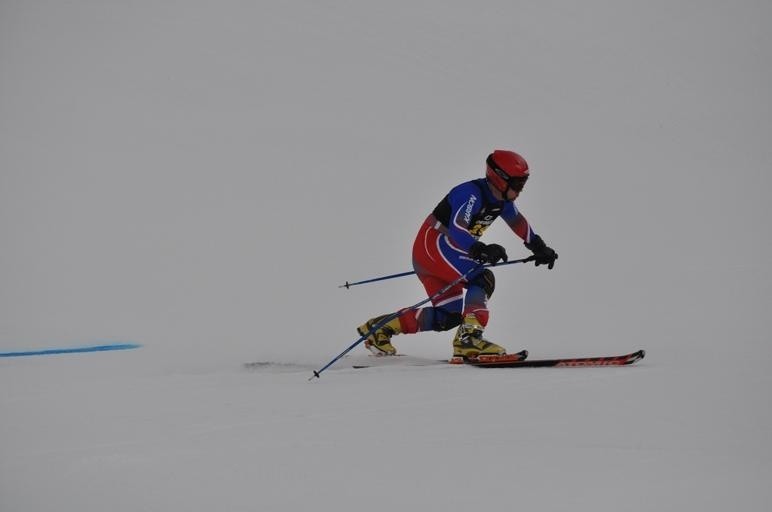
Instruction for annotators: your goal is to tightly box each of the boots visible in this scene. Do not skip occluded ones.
[453,313,505,355]
[357,312,401,354]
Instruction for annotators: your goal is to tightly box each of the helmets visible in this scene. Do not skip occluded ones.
[487,150,529,192]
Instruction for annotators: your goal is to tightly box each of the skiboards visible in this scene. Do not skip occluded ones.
[244,349,645,370]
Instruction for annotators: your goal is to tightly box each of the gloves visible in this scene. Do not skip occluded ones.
[525,234,555,270]
[470,240,507,266]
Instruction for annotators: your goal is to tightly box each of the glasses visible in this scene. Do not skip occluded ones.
[488,154,526,193]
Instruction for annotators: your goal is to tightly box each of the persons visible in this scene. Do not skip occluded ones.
[355,147,559,360]
